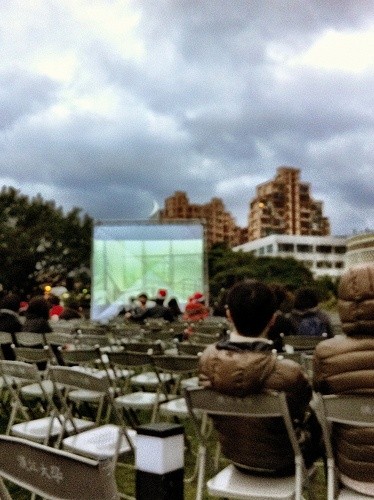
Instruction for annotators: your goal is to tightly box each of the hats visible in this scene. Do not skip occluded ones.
[195,292,205,301]
[292,289,321,315]
[159,290,167,297]
[189,297,195,303]
[20,301,28,307]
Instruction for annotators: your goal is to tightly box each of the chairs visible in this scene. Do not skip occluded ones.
[0,310,374,500]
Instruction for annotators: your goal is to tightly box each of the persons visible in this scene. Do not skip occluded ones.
[311,265,374,497]
[0,294,91,380]
[117,289,227,343]
[264,270,343,353]
[197,281,324,478]
